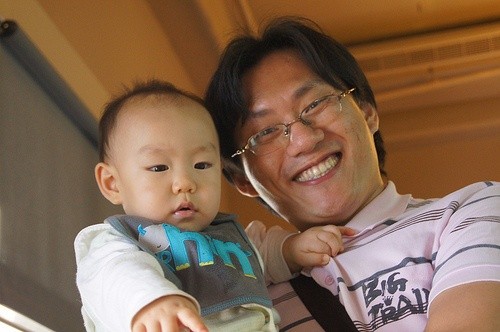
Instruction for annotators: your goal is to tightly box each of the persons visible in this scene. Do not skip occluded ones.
[202,13,500,332]
[73,78,356,332]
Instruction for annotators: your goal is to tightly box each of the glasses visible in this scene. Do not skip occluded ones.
[229,88,360,158]
